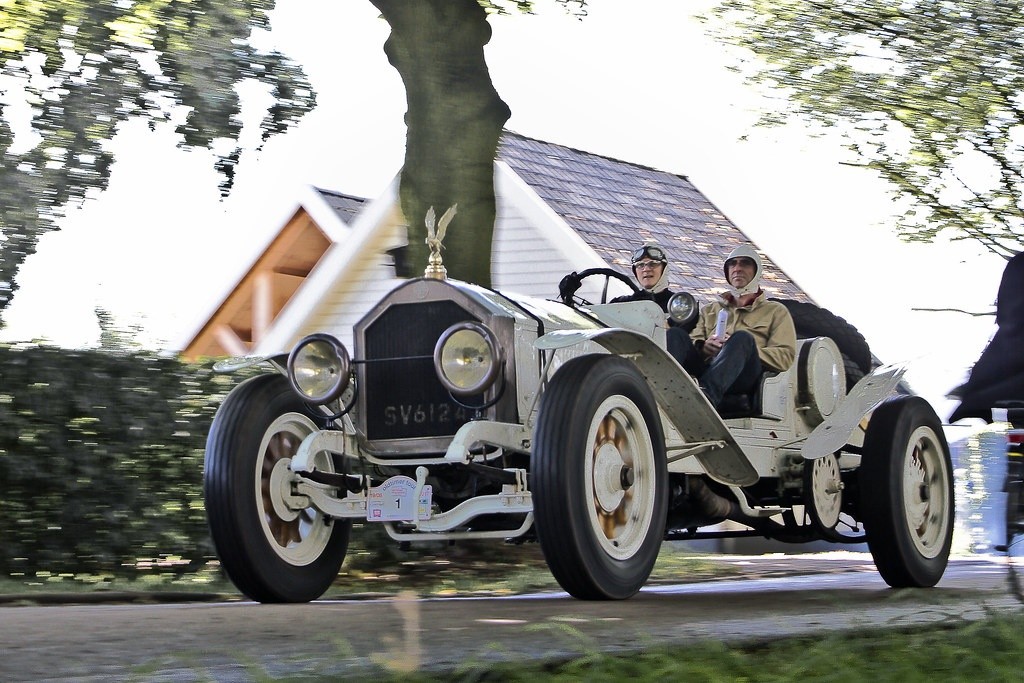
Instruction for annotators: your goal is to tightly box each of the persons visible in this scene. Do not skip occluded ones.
[996,410,1024,552]
[610,242,699,334]
[666,245,796,409]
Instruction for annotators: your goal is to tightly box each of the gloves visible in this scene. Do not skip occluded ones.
[559,272,583,298]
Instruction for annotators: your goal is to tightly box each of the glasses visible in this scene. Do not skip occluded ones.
[725,259,754,268]
[633,259,662,269]
[630,245,667,261]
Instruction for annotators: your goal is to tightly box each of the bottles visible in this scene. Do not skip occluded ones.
[716,308,728,339]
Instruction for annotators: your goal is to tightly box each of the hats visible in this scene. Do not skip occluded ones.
[631,242,671,294]
[721,245,764,296]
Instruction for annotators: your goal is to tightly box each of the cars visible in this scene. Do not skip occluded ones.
[203,265,955,601]
